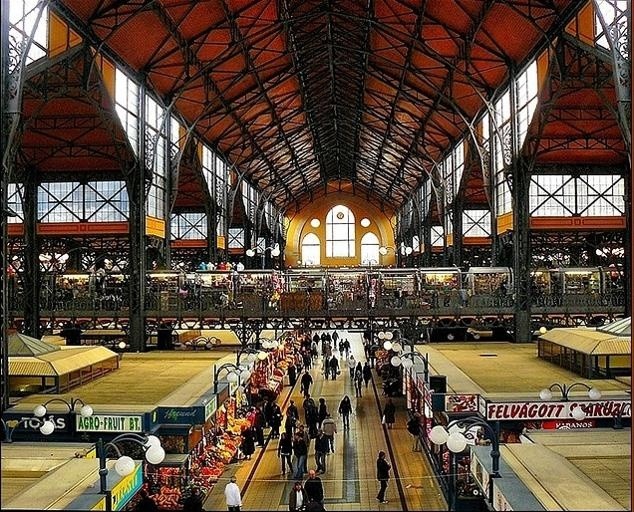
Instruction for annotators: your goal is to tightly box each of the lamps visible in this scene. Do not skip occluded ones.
[214,337,279,394]
[1,396,94,443]
[96,433,168,479]
[540,383,632,429]
[377,329,429,381]
[428,416,501,474]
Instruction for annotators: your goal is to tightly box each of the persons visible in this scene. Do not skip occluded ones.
[223,331,425,511]
[198,259,244,273]
[135,489,158,512]
[182,487,207,511]
